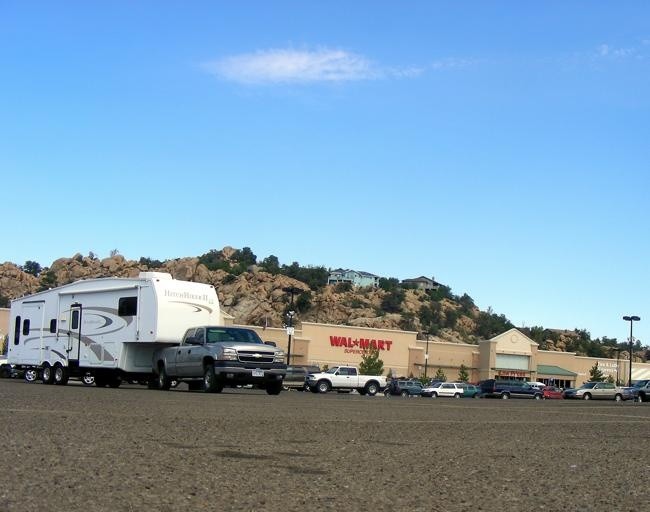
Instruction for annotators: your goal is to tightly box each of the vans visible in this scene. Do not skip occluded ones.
[283,365,319,391]
[623,380,650,402]
[463,384,481,398]
[494,380,544,400]
[421,383,464,399]
[391,380,423,397]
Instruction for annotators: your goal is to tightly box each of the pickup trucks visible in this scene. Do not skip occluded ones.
[304,366,388,395]
[152,326,287,395]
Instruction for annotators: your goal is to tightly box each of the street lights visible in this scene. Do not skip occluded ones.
[283,286,304,365]
[623,315,640,387]
[421,324,431,386]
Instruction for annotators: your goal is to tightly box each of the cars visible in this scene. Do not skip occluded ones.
[565,382,624,401]
[540,386,563,399]
